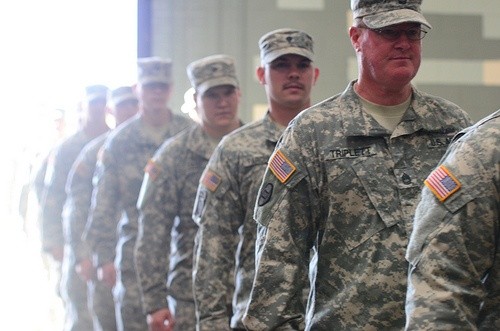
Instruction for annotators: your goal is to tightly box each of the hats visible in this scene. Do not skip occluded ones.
[351,0,432,30]
[259,29,315,63]
[186,55,240,96]
[137,57,172,86]
[112,87,139,107]
[87,85,107,103]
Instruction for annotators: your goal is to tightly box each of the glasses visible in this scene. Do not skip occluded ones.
[358,25,428,40]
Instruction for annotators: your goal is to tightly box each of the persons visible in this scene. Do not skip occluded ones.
[83,59,199,331]
[133,55,250,331]
[190,28,320,331]
[32,84,137,331]
[229,0,473,331]
[404,105,500,331]
